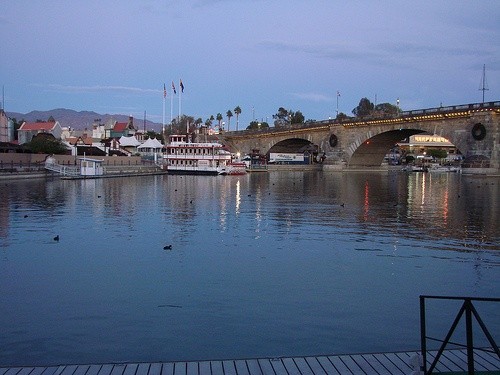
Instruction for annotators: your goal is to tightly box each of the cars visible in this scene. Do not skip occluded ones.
[0,142,129,156]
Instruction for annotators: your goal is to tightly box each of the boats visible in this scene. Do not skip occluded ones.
[162,134,248,176]
[244,148,269,172]
[384,153,463,172]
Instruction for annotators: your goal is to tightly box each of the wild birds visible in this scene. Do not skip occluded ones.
[97,195,102,198]
[53,234,60,242]
[273,184,275,185]
[292,182,296,185]
[340,202,345,207]
[189,199,193,204]
[180,176,182,178]
[163,244,173,251]
[458,194,461,198]
[247,194,251,197]
[470,182,495,188]
[23,214,29,219]
[299,179,302,182]
[268,192,271,195]
[174,189,178,191]
